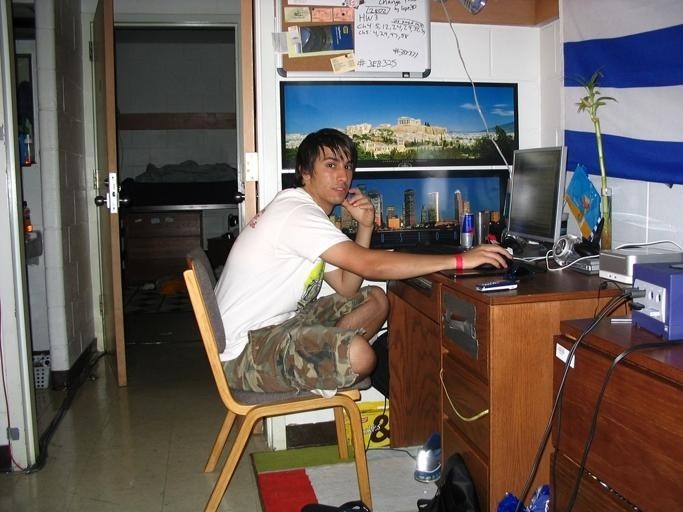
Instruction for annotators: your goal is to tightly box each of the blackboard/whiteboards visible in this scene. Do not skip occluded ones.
[272,0,431,78]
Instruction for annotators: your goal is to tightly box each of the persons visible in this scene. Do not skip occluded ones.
[206,125,514,391]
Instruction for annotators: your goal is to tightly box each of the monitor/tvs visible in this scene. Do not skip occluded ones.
[507,145,568,257]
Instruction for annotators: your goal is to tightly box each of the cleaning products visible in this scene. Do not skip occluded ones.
[18,130,25,165]
[23,200,32,229]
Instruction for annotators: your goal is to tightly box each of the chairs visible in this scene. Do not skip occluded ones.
[182,243,374,512]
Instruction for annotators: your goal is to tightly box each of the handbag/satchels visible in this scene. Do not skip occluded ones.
[301,498,374,512]
[415,452,479,512]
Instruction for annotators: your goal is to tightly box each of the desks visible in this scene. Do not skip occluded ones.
[117,203,239,288]
[385,248,633,512]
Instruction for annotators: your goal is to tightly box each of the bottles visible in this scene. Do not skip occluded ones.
[487,211,504,245]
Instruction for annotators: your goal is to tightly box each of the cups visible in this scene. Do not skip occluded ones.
[475,211,490,246]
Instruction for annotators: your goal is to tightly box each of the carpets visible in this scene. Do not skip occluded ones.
[248,434,441,511]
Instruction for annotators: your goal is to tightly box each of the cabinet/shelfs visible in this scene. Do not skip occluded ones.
[548,334,683,511]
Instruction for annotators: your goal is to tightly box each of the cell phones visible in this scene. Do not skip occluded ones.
[475,281,517,291]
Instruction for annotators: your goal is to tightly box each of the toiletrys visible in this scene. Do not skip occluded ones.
[460,214,475,252]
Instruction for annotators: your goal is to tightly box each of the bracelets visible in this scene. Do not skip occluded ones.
[455,253,464,270]
